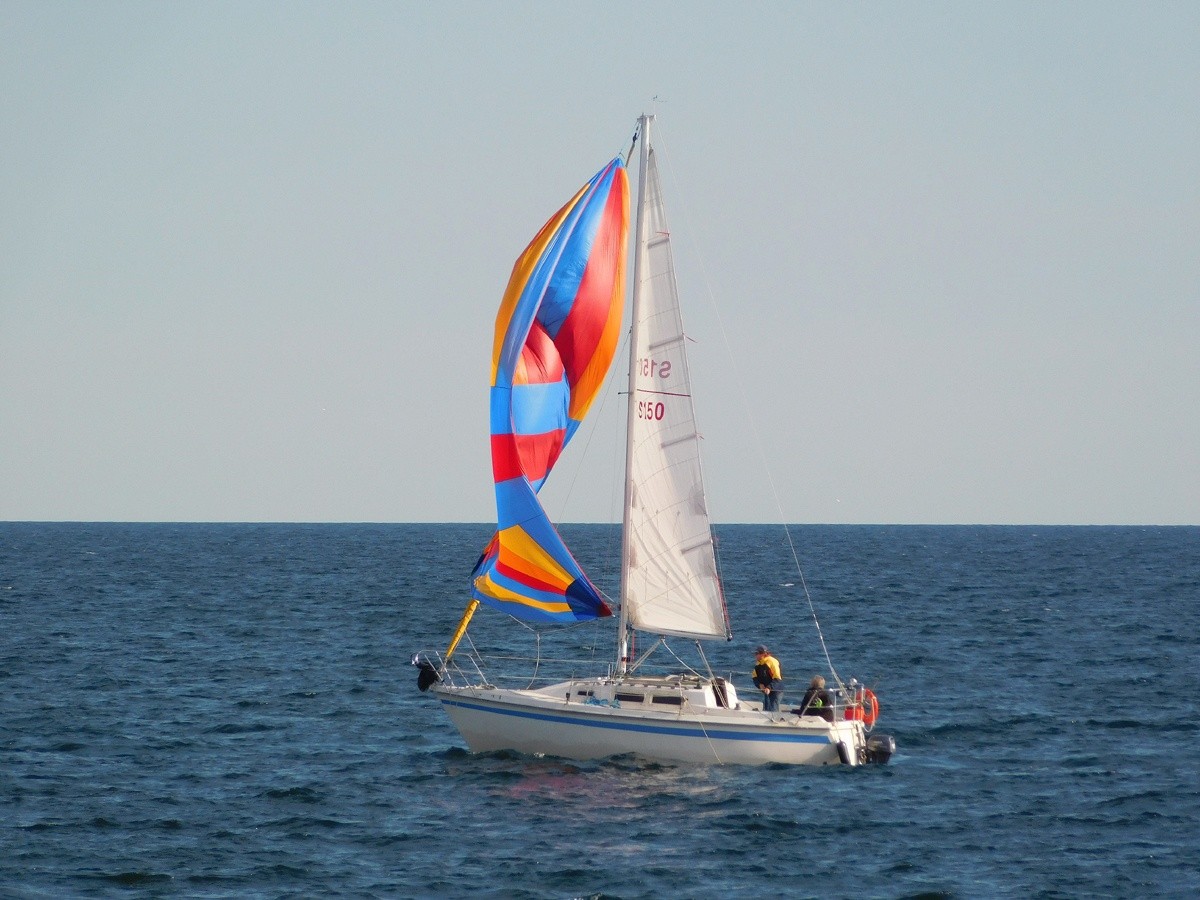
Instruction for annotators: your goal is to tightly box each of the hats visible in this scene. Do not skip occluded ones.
[751,644,767,654]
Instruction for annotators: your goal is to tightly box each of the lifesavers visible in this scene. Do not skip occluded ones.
[856,689,878,724]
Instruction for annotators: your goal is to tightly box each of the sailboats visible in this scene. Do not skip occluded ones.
[411,111,897,769]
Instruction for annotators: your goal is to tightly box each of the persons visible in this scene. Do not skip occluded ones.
[801,676,833,722]
[752,645,784,712]
